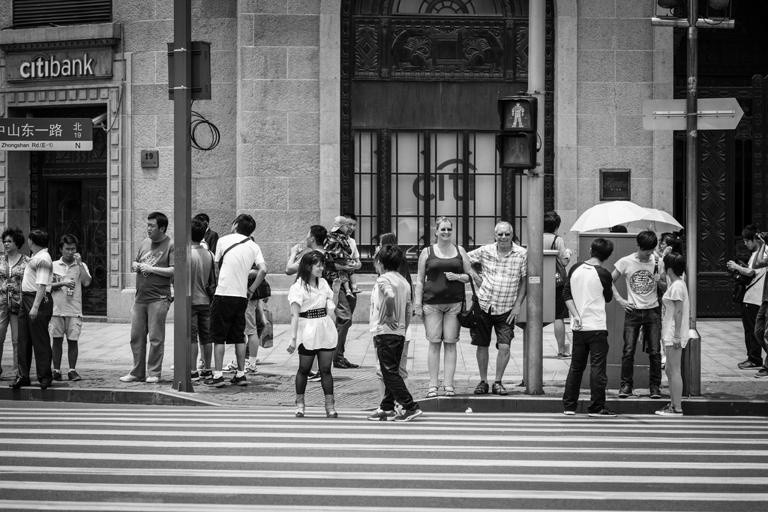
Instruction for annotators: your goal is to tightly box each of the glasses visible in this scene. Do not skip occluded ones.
[497,233,511,236]
[439,228,452,231]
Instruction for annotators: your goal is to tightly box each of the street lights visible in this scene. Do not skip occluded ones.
[657,0,729,399]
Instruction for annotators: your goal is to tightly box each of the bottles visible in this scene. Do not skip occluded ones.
[67,278,75,297]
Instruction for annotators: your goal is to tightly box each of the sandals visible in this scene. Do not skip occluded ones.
[426,386,440,398]
[443,385,457,396]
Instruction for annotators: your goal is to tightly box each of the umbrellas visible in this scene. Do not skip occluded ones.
[625,199,685,236]
[567,195,646,235]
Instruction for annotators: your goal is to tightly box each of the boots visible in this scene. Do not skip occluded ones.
[324,394,339,418]
[293,392,305,417]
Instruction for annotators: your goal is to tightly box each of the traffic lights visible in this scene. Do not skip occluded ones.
[496,96,537,169]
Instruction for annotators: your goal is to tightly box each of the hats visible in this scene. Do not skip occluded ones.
[331,215,347,233]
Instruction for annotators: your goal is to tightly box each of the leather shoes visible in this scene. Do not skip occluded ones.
[40,379,52,390]
[8,378,31,388]
[333,358,359,368]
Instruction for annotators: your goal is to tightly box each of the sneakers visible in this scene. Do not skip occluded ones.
[473,381,490,394]
[754,366,768,378]
[306,370,322,382]
[491,383,508,395]
[395,402,423,422]
[367,408,399,421]
[198,368,213,380]
[50,370,62,381]
[618,383,633,397]
[737,360,764,369]
[221,358,257,373]
[67,370,81,382]
[203,376,225,387]
[146,376,162,382]
[588,407,617,417]
[563,408,576,415]
[119,374,145,383]
[189,371,200,381]
[653,403,684,416]
[229,373,247,386]
[649,385,662,398]
[346,289,356,298]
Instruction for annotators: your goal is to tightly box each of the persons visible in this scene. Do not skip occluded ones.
[8,229,55,391]
[562,237,619,417]
[541,209,573,361]
[48,232,92,384]
[412,212,473,398]
[608,229,662,397]
[651,251,692,418]
[0,228,30,383]
[657,236,687,296]
[466,219,528,396]
[750,232,768,378]
[723,224,765,370]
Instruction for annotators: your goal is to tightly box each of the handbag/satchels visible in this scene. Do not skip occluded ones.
[256,302,275,348]
[655,274,670,315]
[731,268,752,285]
[550,235,567,288]
[247,277,272,301]
[456,272,483,329]
[206,250,218,300]
[732,285,745,303]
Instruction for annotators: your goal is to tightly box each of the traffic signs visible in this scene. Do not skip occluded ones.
[0,117,95,152]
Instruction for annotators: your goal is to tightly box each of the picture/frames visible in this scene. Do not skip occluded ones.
[599,167,633,202]
